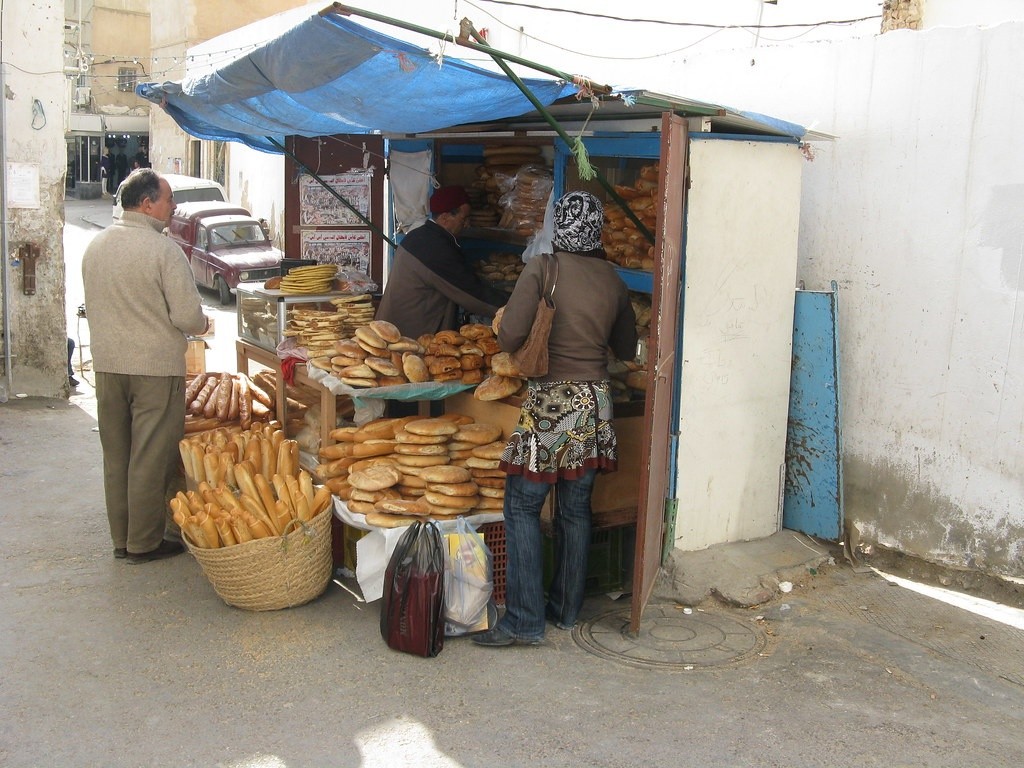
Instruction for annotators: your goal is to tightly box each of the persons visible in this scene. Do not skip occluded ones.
[130,161,140,173]
[91,140,100,182]
[102,147,150,196]
[471,186,637,648]
[80,168,210,564]
[372,186,509,416]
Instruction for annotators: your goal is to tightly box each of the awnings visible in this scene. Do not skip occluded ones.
[136,2,656,251]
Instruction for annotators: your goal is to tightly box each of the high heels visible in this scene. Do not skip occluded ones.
[544,606,564,630]
[471,626,540,646]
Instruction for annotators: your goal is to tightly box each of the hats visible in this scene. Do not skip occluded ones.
[430,185,469,212]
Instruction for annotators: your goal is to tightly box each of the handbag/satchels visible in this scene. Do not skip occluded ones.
[508,254,561,377]
[380,519,444,658]
[433,515,499,636]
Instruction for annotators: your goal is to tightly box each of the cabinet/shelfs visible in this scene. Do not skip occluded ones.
[427,137,667,396]
[235,339,430,494]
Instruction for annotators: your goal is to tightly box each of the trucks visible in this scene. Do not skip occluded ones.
[166,200,286,306]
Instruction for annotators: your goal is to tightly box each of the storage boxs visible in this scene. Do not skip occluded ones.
[237,282,360,354]
[444,390,645,606]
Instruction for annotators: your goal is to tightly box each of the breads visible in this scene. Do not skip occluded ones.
[457,146,552,235]
[169,304,542,550]
[601,293,652,402]
[479,249,528,294]
[597,160,660,272]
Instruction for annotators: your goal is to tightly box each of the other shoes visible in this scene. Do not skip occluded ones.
[112,538,183,564]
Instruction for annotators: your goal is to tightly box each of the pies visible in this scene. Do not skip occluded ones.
[240,265,376,360]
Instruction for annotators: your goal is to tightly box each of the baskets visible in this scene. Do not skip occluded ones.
[180,482,334,612]
